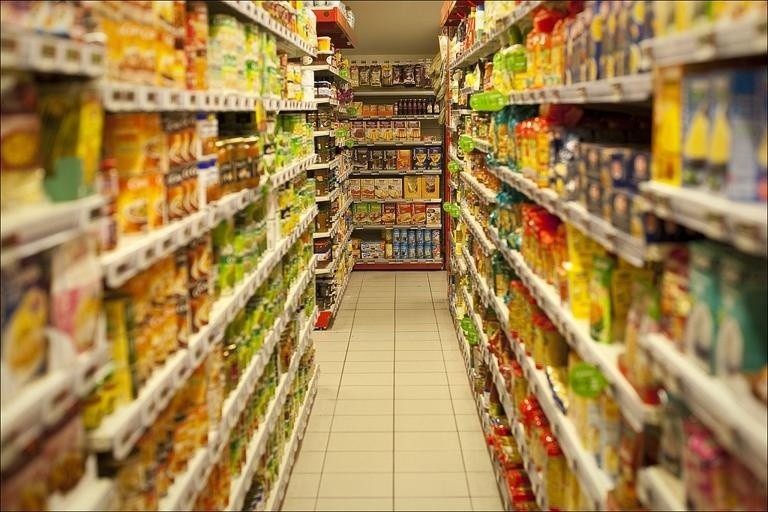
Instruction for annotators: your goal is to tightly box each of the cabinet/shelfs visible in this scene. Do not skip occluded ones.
[440,0,768,512]
[313,0,356,331]
[0,0,321,511]
[346,63,446,271]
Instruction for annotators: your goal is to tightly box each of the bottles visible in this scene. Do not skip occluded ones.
[314,80,337,309]
[394,96,440,115]
[198,115,260,208]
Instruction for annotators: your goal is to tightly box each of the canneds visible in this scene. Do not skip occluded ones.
[215,136,261,196]
